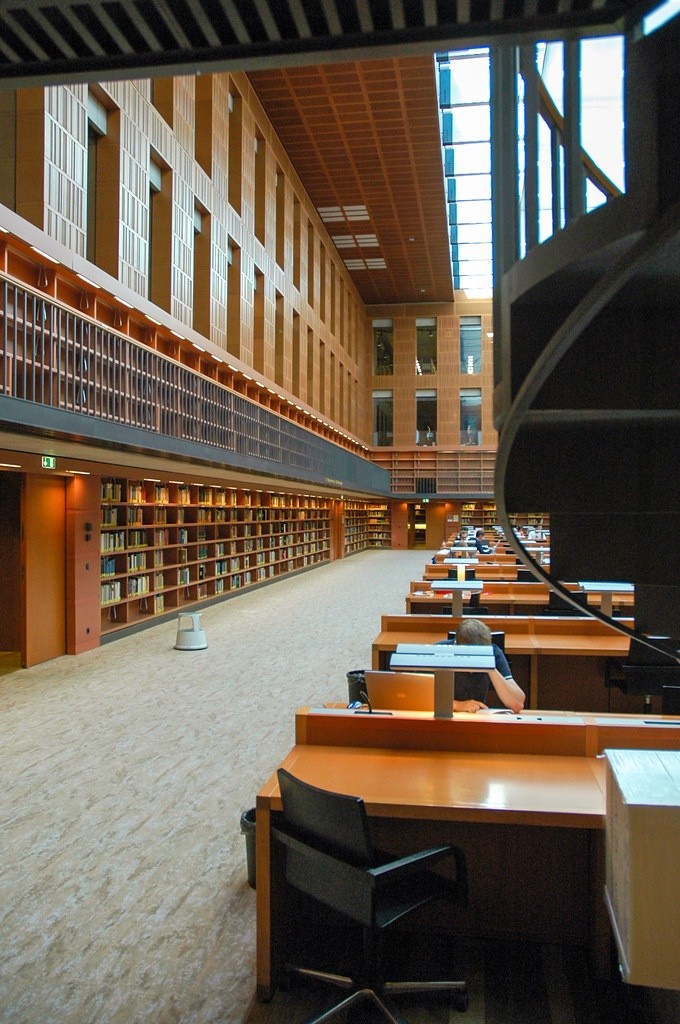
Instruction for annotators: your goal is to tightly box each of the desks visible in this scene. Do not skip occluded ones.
[442,531,550,547]
[405,547,636,615]
[253,614,680,1024]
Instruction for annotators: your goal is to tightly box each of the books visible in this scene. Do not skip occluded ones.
[100,483,389,607]
[460,504,543,524]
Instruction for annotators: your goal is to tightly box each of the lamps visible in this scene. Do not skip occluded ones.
[578,578,637,619]
[430,580,485,618]
[387,639,499,718]
[441,558,481,581]
[449,545,478,558]
[535,528,550,539]
[525,546,551,565]
[454,526,535,543]
[519,540,537,547]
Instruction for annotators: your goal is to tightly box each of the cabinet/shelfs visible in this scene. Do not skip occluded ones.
[93,475,253,639]
[368,446,499,498]
[413,505,427,542]
[603,747,680,996]
[253,483,335,584]
[340,498,392,557]
[459,502,549,531]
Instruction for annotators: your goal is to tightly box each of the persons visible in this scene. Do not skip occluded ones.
[527,524,547,540]
[454,527,493,554]
[514,525,523,536]
[434,619,527,713]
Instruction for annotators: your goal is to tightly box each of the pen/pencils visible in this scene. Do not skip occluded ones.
[517,717,541,720]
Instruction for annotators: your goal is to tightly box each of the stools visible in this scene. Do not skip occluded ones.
[170,609,210,651]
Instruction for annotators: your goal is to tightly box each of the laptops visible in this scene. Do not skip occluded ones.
[365,669,434,711]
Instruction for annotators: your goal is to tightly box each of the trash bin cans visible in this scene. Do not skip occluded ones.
[346,670,372,704]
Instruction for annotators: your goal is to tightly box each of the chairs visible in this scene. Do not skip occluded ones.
[266,766,472,1024]
[598,624,680,723]
